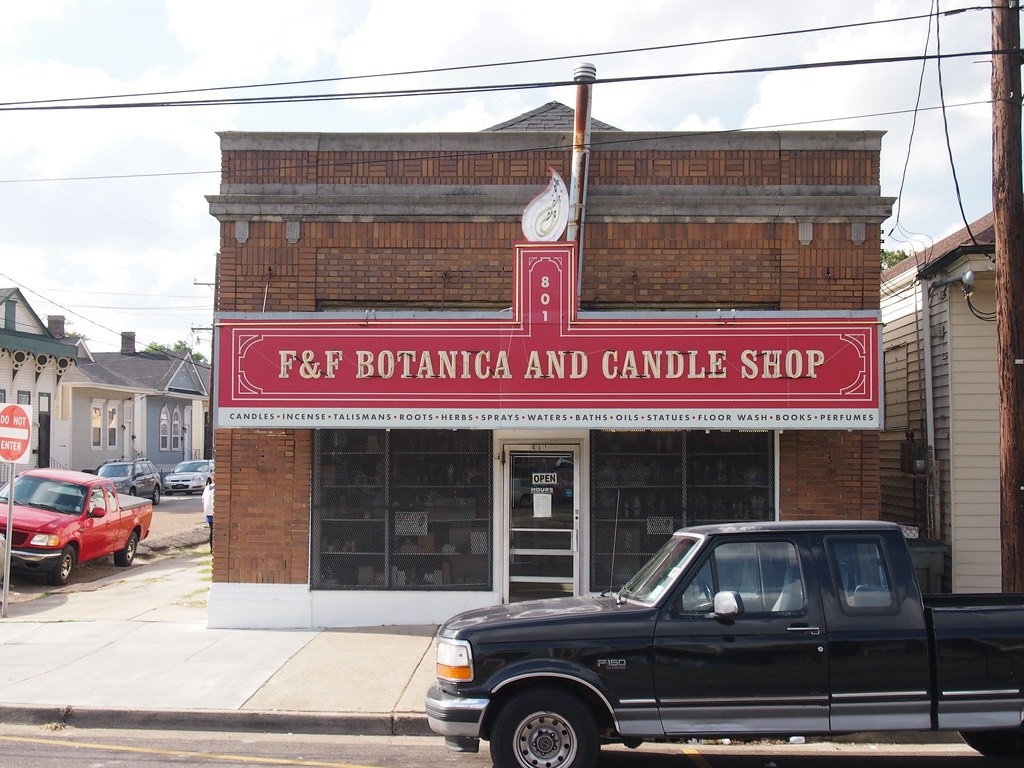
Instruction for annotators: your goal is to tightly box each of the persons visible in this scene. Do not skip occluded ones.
[201,471,215,553]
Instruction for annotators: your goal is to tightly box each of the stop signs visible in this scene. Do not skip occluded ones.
[0,404,31,461]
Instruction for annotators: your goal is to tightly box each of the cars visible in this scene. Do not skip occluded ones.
[163,460,214,496]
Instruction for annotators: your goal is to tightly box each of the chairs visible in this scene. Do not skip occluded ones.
[769,566,804,611]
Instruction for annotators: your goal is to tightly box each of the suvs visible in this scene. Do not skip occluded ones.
[98,458,162,506]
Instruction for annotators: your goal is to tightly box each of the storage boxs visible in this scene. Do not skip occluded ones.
[449,528,487,552]
[358,567,375,585]
[425,498,475,520]
[419,532,435,553]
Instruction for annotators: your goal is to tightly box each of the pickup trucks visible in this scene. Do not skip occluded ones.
[0,468,153,586]
[423,520,1024,768]
